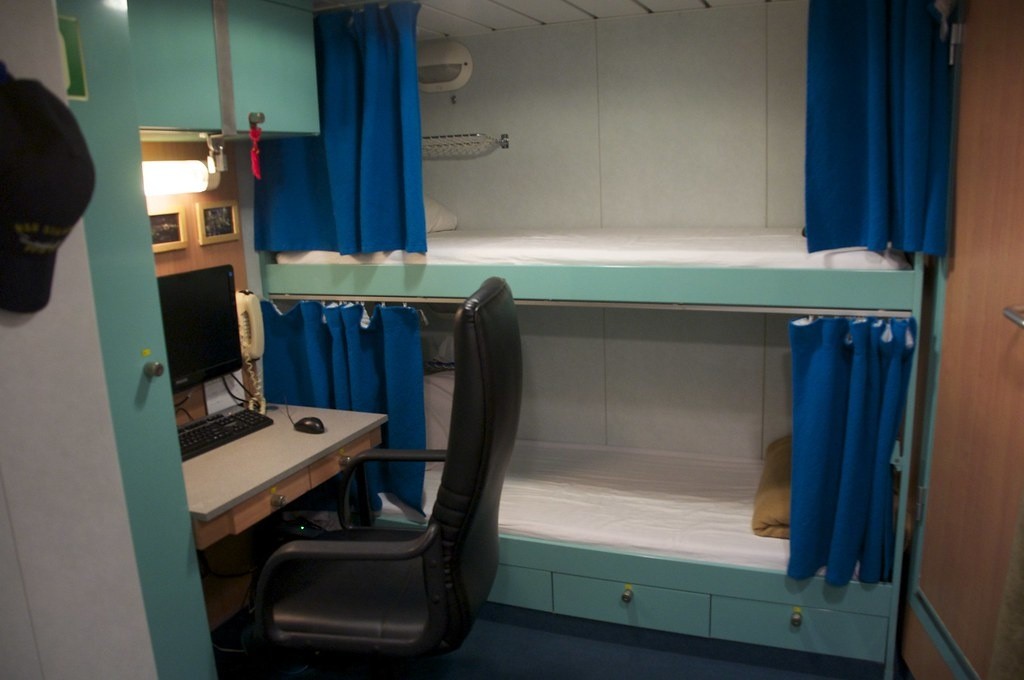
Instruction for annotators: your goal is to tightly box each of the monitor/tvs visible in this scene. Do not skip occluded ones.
[159,264,243,395]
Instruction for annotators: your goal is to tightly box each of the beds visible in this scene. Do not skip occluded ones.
[257,234,929,679]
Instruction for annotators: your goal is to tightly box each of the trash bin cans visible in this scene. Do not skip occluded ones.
[211,604,271,680]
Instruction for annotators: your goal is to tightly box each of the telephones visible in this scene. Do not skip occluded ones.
[235,290,265,362]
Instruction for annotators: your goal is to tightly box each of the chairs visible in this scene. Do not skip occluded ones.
[252,275,525,680]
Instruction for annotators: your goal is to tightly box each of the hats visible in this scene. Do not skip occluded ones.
[0,63,96,313]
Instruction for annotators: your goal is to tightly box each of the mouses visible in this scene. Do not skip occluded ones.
[293,417,324,434]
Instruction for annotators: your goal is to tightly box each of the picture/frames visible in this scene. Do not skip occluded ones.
[147,205,188,255]
[196,198,241,246]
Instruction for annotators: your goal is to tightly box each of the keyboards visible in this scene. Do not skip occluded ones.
[178,405,273,461]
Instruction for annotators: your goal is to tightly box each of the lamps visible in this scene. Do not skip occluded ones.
[141,130,230,197]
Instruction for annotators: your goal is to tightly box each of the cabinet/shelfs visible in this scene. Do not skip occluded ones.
[127,0,320,143]
[891,0,1024,679]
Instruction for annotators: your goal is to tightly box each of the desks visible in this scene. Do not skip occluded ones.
[178,398,391,636]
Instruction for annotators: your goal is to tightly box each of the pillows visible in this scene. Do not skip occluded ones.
[423,197,458,231]
[424,370,455,470]
